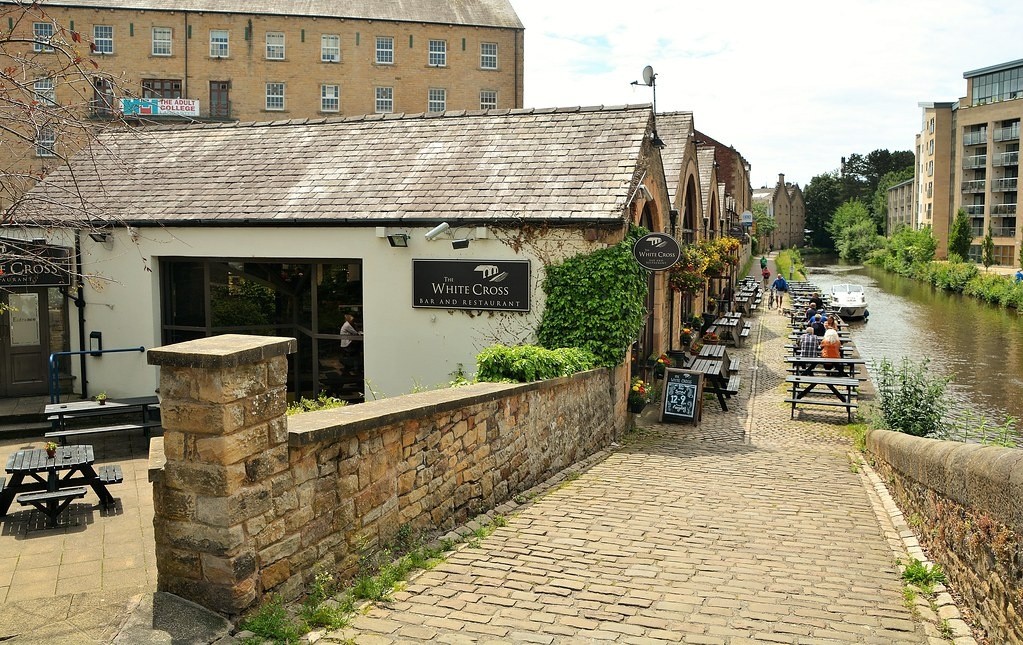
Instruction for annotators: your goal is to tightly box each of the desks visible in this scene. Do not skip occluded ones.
[0,445,114,527]
[736,292,753,297]
[790,303,832,307]
[690,359,723,376]
[44,396,162,446]
[742,286,756,292]
[785,375,860,387]
[724,312,742,318]
[792,298,832,303]
[788,322,849,327]
[787,315,839,320]
[790,308,841,314]
[792,328,850,334]
[713,317,738,327]
[786,356,865,364]
[787,334,852,342]
[784,344,853,352]
[733,297,748,302]
[699,345,726,358]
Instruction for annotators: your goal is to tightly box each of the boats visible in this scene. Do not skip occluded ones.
[829,284,868,317]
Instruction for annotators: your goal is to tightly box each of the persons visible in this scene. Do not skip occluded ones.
[805,292,839,336]
[820,328,844,377]
[760,256,771,292]
[770,273,789,309]
[339,314,363,357]
[798,327,820,376]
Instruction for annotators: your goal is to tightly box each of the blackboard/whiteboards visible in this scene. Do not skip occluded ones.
[659,367,703,423]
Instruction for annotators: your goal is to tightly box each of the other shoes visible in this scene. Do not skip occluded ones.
[765,288,768,291]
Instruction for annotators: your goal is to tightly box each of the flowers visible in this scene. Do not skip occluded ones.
[96,393,107,400]
[692,313,704,324]
[669,234,740,307]
[629,379,654,403]
[656,353,672,372]
[43,441,57,450]
[682,328,694,340]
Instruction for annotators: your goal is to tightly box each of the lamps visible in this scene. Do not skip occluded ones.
[88,232,111,242]
[425,221,450,241]
[649,130,666,149]
[452,238,469,249]
[713,160,720,167]
[691,139,707,146]
[387,232,410,247]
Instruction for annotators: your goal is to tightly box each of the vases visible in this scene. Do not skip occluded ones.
[694,327,700,331]
[654,373,665,379]
[47,450,55,457]
[710,307,715,311]
[682,340,690,346]
[630,402,645,414]
[99,400,106,405]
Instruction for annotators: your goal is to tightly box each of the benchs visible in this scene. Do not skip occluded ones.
[684,276,763,395]
[784,279,867,408]
[47,407,157,421]
[17,487,87,505]
[45,421,161,441]
[99,465,123,485]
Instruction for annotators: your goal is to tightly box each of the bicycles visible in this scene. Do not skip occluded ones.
[768,289,777,310]
